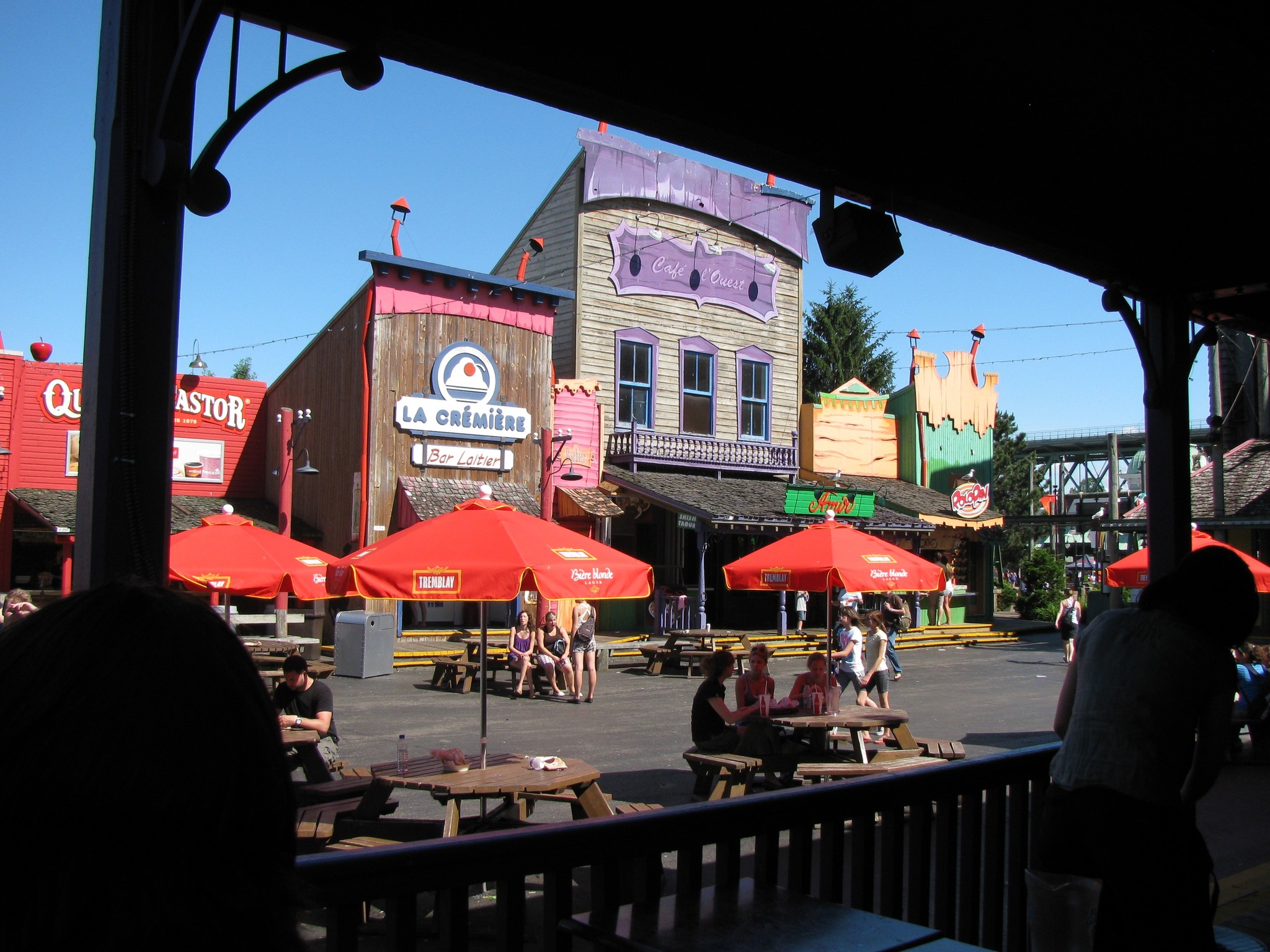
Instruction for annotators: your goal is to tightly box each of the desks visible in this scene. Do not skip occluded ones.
[281,723,336,782]
[240,638,302,692]
[334,753,616,838]
[444,636,568,691]
[642,628,772,679]
[570,876,942,952]
[898,936,998,952]
[690,703,927,803]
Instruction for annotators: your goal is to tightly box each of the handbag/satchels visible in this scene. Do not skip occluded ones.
[1010,574,1016,581]
[1059,599,1076,630]
[553,638,566,657]
[1004,572,1008,579]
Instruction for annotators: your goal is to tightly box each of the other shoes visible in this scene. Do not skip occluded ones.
[894,673,903,680]
[568,698,581,704]
[1062,655,1069,663]
[874,726,884,736]
[553,691,565,696]
[795,630,807,635]
[515,689,523,696]
[569,692,583,697]
[529,694,536,699]
[584,698,592,703]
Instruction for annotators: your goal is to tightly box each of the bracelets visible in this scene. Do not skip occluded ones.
[752,703,760,712]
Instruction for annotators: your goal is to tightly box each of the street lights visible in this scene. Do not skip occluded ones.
[528,426,584,629]
[275,407,320,638]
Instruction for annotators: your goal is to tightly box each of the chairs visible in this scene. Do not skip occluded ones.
[647,599,689,633]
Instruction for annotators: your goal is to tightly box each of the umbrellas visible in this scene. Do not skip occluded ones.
[324,485,654,769]
[166,503,357,635]
[1102,521,1270,594]
[723,509,946,714]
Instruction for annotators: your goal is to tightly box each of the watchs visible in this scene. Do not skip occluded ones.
[295,716,302,726]
[890,608,894,613]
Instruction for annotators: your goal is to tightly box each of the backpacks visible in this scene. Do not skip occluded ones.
[891,594,912,632]
[575,605,595,644]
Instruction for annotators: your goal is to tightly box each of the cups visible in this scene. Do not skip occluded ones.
[173,447,180,474]
[824,685,841,715]
[707,623,711,632]
[812,692,823,715]
[184,461,204,478]
[199,454,221,479]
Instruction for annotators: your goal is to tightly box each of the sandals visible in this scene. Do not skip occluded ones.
[864,738,873,742]
[874,740,883,744]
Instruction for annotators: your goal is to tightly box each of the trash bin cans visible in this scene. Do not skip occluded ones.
[264,595,326,660]
[333,609,395,679]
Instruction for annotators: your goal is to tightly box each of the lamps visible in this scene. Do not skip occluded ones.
[801,480,825,500]
[834,483,858,505]
[864,485,888,508]
[695,228,723,256]
[754,244,777,276]
[188,338,208,375]
[636,212,664,243]
[811,188,905,280]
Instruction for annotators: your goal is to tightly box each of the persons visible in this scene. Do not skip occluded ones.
[408,600,427,630]
[688,588,910,791]
[0,587,41,626]
[993,565,1270,952]
[270,655,340,783]
[0,578,302,952]
[508,609,536,699]
[568,600,597,704]
[536,611,583,697]
[928,552,953,626]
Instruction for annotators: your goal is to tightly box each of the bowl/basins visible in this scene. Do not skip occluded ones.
[442,761,470,772]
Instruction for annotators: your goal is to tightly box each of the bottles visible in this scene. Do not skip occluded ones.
[528,756,544,771]
[759,694,771,717]
[803,682,812,708]
[397,734,409,771]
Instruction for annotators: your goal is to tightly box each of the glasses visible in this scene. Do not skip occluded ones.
[838,613,848,618]
[751,646,765,653]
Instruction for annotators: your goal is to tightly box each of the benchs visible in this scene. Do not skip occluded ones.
[251,654,338,697]
[682,728,966,832]
[292,755,665,924]
[429,652,576,695]
[638,641,778,680]
